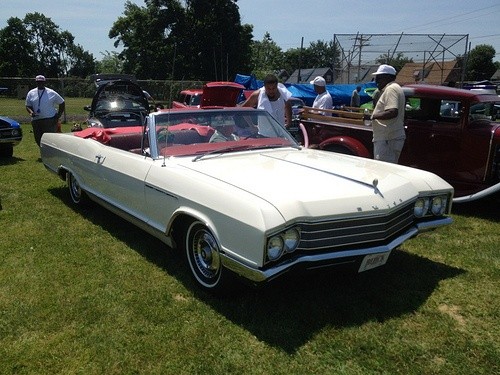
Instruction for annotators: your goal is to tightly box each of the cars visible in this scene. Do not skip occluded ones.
[83,79,158,129]
[171,81,259,141]
[455,80,500,109]
[0,114,23,159]
[284,83,500,211]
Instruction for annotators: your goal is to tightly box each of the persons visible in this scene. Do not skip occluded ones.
[238,74,292,139]
[141,87,155,105]
[447,80,456,88]
[326,72,331,82]
[24,75,65,160]
[370,64,406,164]
[309,76,333,122]
[372,87,382,107]
[350,86,361,118]
[209,115,240,145]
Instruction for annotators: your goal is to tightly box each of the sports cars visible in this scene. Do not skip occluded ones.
[40,105,455,296]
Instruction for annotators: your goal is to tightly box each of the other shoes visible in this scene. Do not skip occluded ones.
[38,157,42,161]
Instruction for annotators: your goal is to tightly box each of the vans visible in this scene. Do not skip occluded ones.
[90,73,136,86]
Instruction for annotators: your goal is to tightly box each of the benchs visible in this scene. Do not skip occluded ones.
[105,129,207,151]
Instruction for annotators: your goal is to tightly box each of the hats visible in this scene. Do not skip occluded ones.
[309,76,326,86]
[35,75,46,81]
[371,64,397,76]
